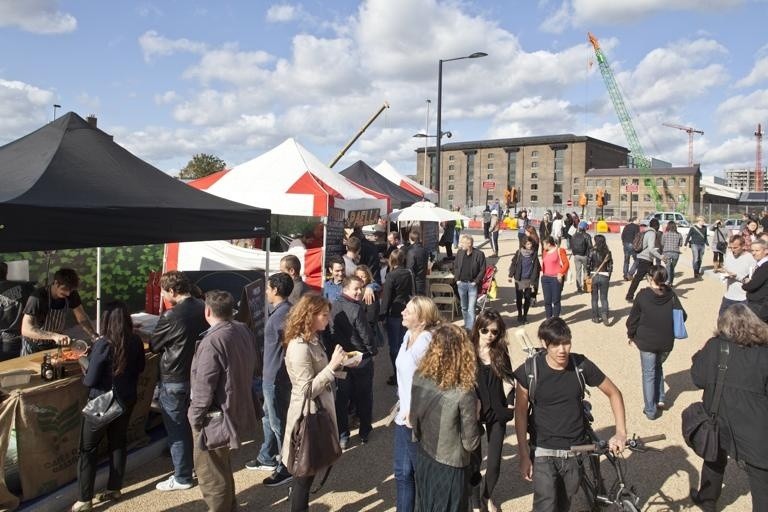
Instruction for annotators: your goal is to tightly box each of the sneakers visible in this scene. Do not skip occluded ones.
[601,313,609,326]
[263,462,293,487]
[156,475,193,490]
[245,459,278,472]
[340,436,351,450]
[359,438,369,445]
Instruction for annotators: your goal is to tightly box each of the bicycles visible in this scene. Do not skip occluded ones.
[570,427,666,512]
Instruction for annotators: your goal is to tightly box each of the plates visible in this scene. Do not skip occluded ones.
[339,350,364,365]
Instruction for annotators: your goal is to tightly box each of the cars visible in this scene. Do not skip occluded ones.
[724,219,745,230]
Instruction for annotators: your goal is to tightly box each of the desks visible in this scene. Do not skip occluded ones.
[423,271,457,283]
[1,341,177,507]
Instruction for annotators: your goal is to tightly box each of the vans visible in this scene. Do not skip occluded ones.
[640,212,690,227]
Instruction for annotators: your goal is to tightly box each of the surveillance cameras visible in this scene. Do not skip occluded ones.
[448,133,452,138]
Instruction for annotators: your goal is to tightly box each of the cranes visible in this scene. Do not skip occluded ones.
[661,121,704,168]
[754,123,764,191]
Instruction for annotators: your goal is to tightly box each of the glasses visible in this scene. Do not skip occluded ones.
[646,273,652,279]
[478,327,498,335]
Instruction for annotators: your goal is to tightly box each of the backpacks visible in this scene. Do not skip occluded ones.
[632,229,657,254]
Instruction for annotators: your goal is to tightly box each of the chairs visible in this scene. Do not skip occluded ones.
[430,283,462,323]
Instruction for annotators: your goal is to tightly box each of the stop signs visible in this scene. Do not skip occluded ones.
[567,200,572,207]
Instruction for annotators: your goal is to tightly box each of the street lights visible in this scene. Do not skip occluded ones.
[412,50,489,211]
[53,103,61,121]
[421,97,432,185]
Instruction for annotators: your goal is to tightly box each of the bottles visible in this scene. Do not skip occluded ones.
[40,352,62,380]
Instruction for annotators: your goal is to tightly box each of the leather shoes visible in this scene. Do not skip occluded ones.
[95,489,123,502]
[65,500,94,512]
[689,488,714,512]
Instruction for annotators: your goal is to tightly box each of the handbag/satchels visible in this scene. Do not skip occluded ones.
[672,308,688,338]
[583,278,593,293]
[286,382,343,478]
[82,386,125,434]
[681,401,720,463]
[716,242,726,253]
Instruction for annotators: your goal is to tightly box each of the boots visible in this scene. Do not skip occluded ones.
[713,260,718,273]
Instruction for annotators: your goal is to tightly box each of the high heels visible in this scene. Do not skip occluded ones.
[481,494,498,512]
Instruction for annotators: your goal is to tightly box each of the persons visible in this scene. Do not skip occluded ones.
[245,271,295,488]
[67,303,147,512]
[19,266,102,355]
[146,268,207,493]
[182,285,259,512]
[0,260,36,362]
[279,206,767,512]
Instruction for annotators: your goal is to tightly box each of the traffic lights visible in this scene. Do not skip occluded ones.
[507,191,510,203]
[513,189,517,202]
[600,191,605,206]
